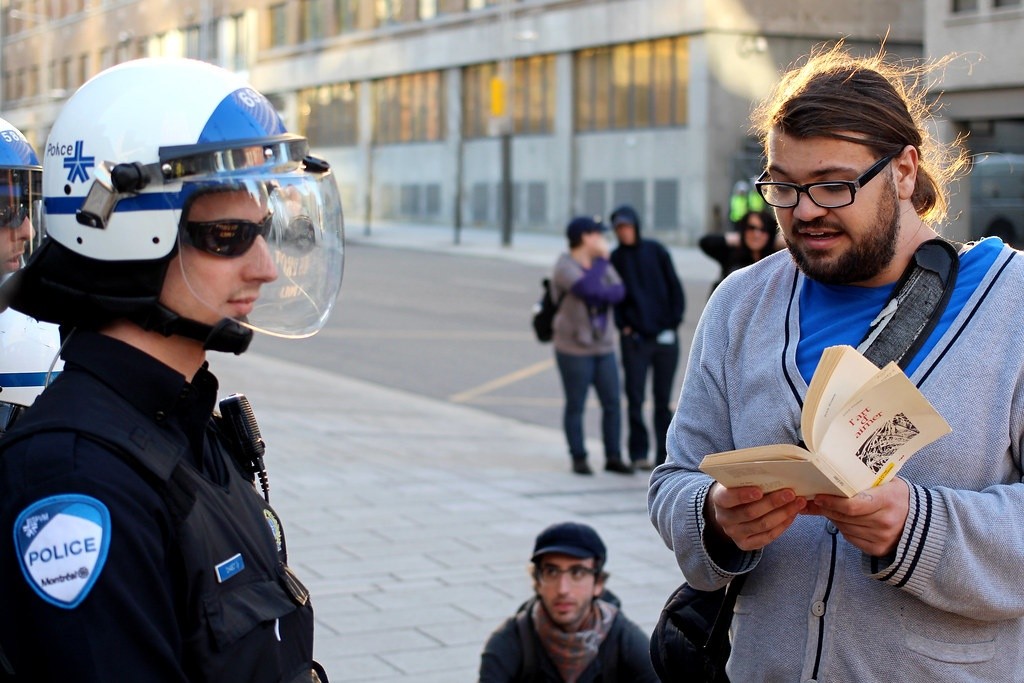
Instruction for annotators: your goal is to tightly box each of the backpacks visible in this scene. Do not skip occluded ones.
[532,279,565,343]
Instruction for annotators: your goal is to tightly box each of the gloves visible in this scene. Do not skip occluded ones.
[657,329,676,344]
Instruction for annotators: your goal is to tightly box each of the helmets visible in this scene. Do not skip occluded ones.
[42,59,331,262]
[0,117,43,200]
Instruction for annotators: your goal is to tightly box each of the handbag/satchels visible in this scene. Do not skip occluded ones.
[650,581,731,683]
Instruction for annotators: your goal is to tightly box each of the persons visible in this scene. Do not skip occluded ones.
[479,522,660,683]
[609,207,685,472]
[730,175,765,223]
[647,56,1024,683]
[0,118,43,287]
[551,217,635,476]
[0,56,347,683]
[699,212,788,280]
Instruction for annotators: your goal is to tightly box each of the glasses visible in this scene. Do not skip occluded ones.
[2,203,27,230]
[748,225,768,233]
[755,154,891,208]
[179,217,316,259]
[539,564,596,579]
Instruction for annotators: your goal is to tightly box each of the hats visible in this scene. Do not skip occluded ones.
[736,180,749,191]
[613,212,635,228]
[567,217,608,239]
[532,522,607,567]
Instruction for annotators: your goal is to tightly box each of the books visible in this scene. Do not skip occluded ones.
[699,345,954,500]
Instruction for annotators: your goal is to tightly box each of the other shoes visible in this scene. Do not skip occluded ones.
[605,460,634,475]
[630,447,650,461]
[574,460,593,477]
